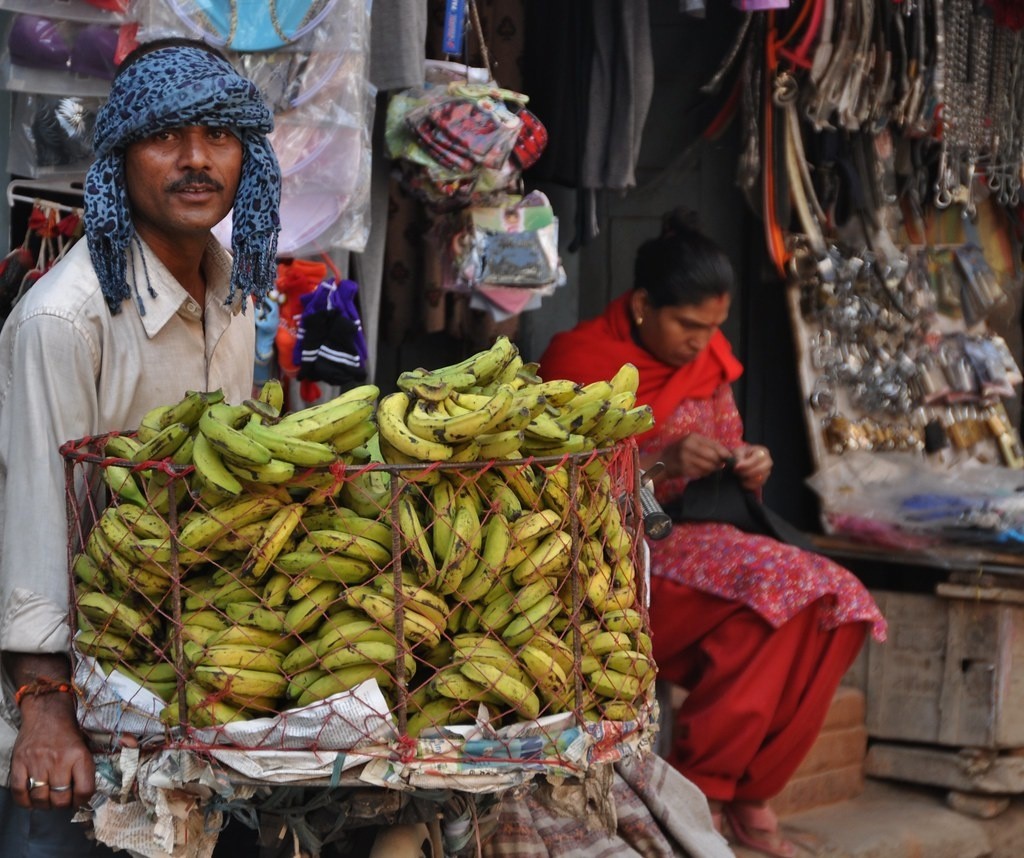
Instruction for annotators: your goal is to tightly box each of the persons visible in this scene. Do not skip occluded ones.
[0,38,283,858]
[540,224,888,858]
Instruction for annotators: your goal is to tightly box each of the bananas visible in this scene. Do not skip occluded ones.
[73,333,659,743]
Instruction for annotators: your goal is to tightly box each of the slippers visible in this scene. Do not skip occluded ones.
[713,813,722,835]
[724,799,797,858]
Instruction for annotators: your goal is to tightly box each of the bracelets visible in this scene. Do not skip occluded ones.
[15,669,73,707]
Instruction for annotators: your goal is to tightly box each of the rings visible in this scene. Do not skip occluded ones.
[28,777,47,791]
[50,784,71,792]
[758,450,765,458]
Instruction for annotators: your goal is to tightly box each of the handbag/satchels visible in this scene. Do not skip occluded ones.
[253,259,367,384]
